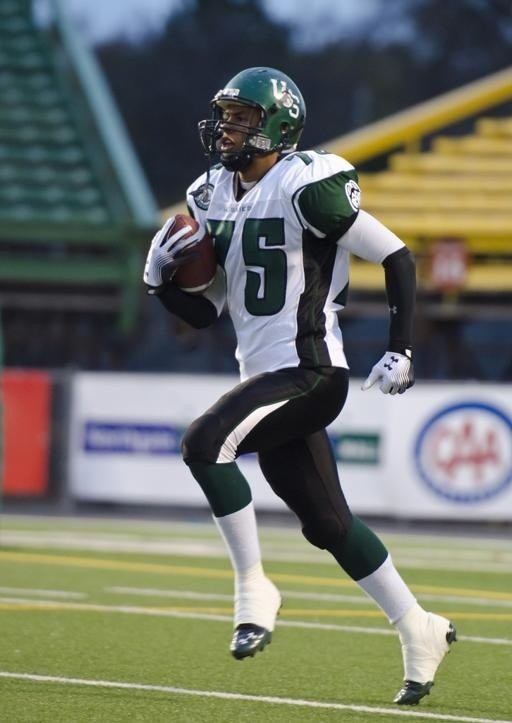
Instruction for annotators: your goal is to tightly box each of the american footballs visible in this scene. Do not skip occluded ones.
[164,213,217,296]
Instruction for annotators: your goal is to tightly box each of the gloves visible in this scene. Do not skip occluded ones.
[360,349,414,395]
[142,217,201,296]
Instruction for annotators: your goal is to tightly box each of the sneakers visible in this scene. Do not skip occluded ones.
[393,617,456,705]
[229,583,283,661]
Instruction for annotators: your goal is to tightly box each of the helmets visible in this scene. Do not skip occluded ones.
[197,67,308,172]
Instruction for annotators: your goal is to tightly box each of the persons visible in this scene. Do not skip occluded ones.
[140,65,460,706]
